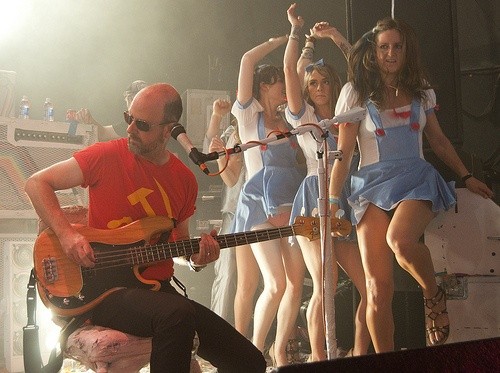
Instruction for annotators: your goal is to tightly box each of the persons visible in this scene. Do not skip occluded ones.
[23,82,268,373]
[66,79,150,139]
[205,83,247,330]
[230,26,317,369]
[282,0,371,364]
[327,17,494,357]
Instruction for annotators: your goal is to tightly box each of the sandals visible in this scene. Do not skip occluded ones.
[424,286,450,347]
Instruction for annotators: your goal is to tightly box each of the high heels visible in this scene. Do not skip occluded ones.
[269,343,290,368]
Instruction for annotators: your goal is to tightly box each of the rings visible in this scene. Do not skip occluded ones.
[206,252,211,255]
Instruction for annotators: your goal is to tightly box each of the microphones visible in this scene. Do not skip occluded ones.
[169,123,210,175]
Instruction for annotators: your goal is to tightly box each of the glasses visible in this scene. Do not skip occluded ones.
[123,111,176,131]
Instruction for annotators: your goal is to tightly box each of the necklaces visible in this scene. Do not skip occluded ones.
[373,75,401,97]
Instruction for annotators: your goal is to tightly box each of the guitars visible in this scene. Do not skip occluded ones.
[33,209,351,317]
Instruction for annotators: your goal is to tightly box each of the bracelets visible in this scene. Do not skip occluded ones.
[306,36,317,48]
[327,197,343,206]
[300,47,314,61]
[461,172,475,184]
[288,35,300,42]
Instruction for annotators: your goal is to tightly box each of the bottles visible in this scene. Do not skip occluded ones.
[20,96,30,120]
[43,97,54,122]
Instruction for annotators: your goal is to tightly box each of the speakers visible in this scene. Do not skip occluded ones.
[270,336,500,373]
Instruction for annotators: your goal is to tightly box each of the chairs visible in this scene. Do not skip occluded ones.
[38,205,203,373]
